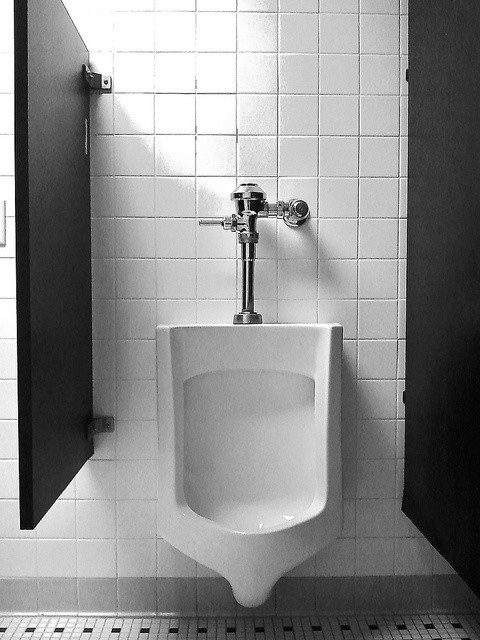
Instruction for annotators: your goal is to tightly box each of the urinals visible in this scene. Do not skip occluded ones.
[156,323,342,610]
